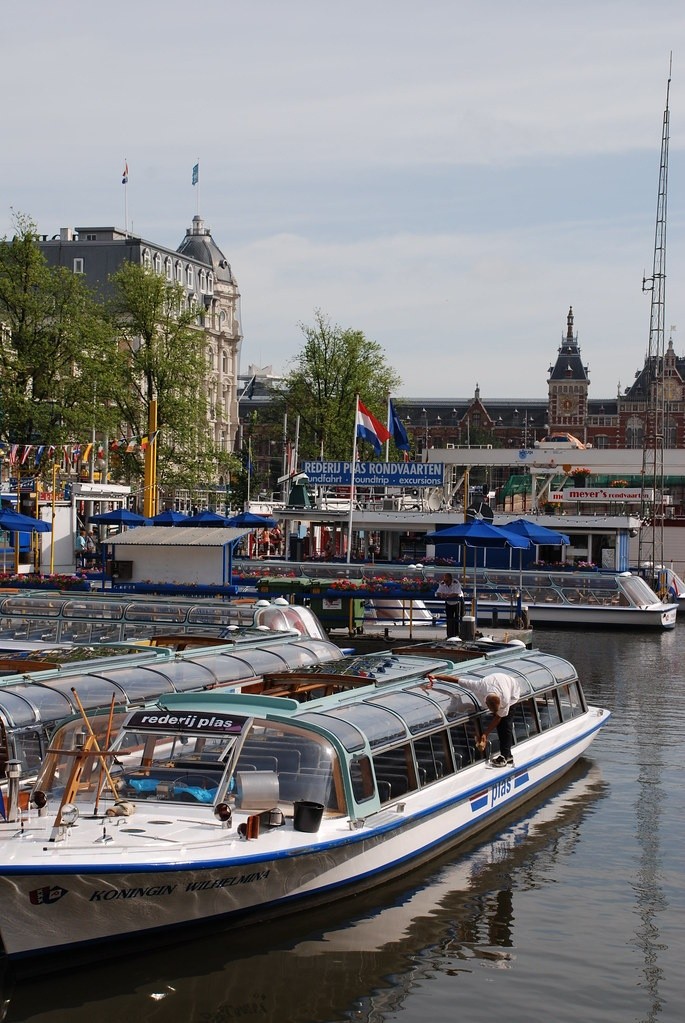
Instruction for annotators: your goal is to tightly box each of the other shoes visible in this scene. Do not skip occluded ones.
[493,755,514,767]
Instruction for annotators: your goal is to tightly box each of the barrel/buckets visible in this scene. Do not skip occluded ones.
[294,801,324,833]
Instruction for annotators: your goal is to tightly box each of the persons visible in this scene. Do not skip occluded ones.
[434,573,466,640]
[252,525,282,554]
[76,530,95,568]
[428,673,520,766]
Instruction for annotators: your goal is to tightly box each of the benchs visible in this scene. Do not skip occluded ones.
[0,674,581,817]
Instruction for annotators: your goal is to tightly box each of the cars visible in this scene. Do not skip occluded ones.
[534,432,585,450]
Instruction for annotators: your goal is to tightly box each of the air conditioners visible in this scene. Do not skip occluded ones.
[383,499,401,512]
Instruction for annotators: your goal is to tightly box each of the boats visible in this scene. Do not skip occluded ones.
[0,529,684,987]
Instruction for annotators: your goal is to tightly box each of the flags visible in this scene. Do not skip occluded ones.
[122,164,128,184]
[247,449,252,477]
[192,164,198,184]
[357,400,390,457]
[0,429,159,465]
[390,400,411,451]
[247,377,255,399]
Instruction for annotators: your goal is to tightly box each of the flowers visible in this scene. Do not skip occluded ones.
[0,571,88,587]
[330,575,437,592]
[312,552,358,560]
[536,559,595,567]
[230,567,295,578]
[421,556,453,564]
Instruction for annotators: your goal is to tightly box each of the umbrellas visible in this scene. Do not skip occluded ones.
[179,510,236,527]
[149,510,189,527]
[0,508,51,574]
[88,509,154,532]
[232,512,275,556]
[425,519,530,598]
[500,519,569,589]
